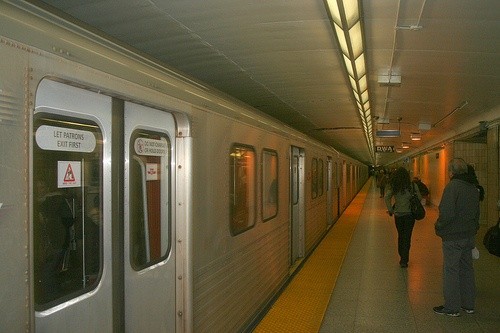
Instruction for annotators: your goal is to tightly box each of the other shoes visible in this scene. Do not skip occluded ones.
[462,305,473,314]
[433,305,461,316]
[401,262,409,267]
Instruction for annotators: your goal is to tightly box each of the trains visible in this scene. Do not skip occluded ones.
[0,0,371,333]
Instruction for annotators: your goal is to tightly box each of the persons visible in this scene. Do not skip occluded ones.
[386,169,427,269]
[412,174,431,208]
[376,168,389,200]
[433,157,476,317]
[33,178,101,305]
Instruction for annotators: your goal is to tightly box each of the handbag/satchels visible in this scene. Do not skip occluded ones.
[409,181,426,219]
[482,215,500,257]
